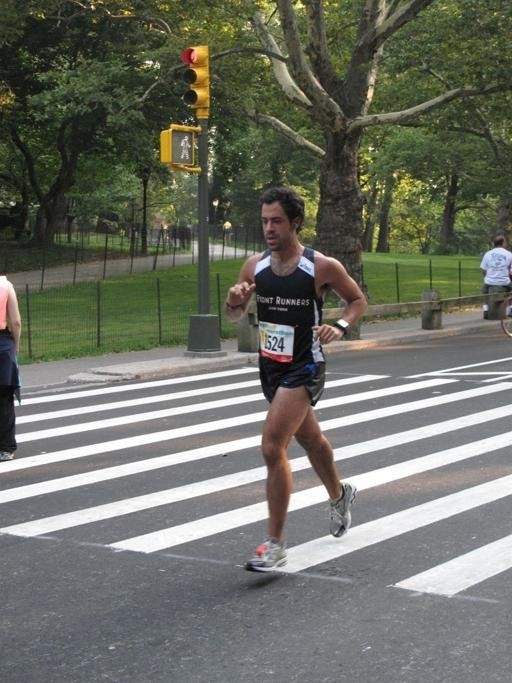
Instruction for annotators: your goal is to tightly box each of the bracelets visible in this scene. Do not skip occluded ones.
[223,296,242,312]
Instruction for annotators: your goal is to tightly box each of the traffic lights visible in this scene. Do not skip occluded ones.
[161,124,196,167]
[181,45,210,111]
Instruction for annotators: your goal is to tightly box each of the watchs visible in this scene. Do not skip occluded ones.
[333,318,350,336]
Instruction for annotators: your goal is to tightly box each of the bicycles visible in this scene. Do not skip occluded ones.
[499,295,512,337]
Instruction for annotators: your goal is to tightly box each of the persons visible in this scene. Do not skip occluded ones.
[222,218,232,245]
[221,185,368,574]
[0,274,24,462]
[478,234,512,319]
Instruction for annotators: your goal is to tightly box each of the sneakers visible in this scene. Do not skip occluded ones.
[1,452,14,461]
[508,308,512,317]
[245,537,289,573]
[328,481,357,537]
[484,311,489,319]
